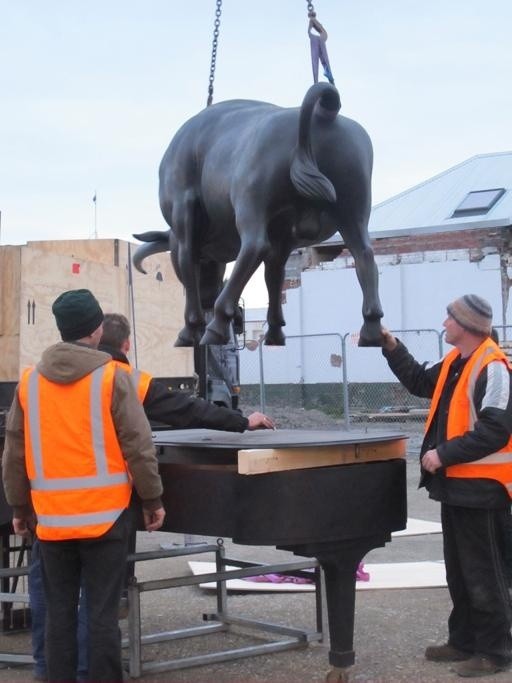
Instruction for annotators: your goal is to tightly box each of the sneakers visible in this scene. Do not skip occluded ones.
[425,645,506,676]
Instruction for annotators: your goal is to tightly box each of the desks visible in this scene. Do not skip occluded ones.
[0,430,410,681]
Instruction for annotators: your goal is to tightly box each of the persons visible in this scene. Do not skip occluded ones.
[490,328,498,345]
[381,294,512,677]
[3,291,165,683]
[28,314,275,683]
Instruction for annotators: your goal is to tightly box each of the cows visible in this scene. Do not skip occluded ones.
[129,78,394,349]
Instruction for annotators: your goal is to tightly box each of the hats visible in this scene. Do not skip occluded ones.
[446,294,492,334]
[52,289,103,342]
